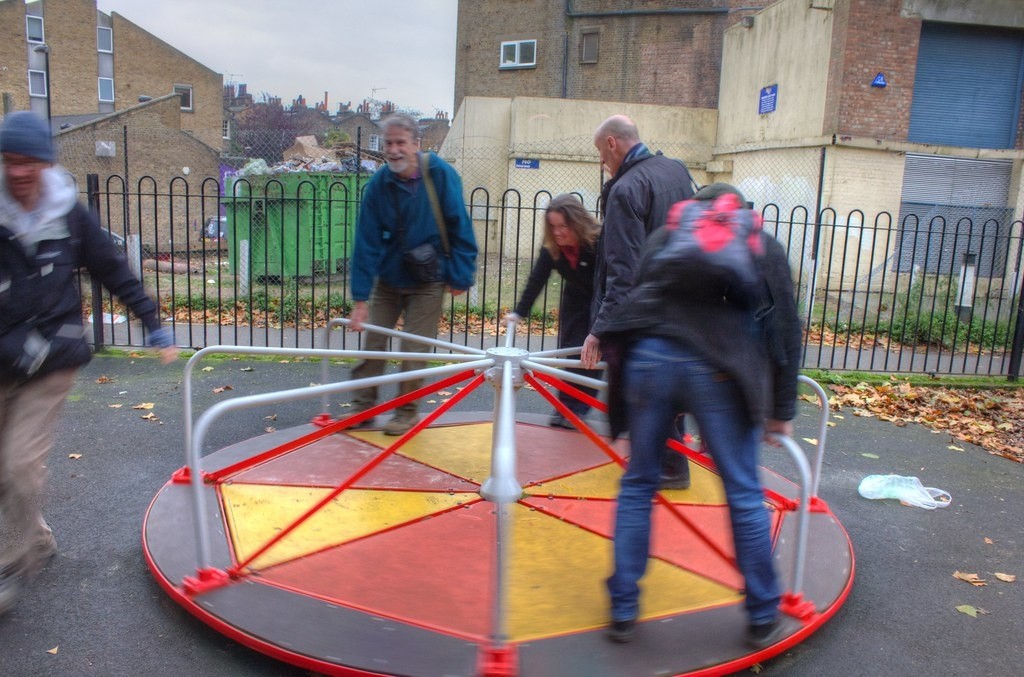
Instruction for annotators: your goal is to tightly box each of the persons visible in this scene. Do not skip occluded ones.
[596,182,805,649]
[348,111,480,436]
[580,115,701,491]
[1,111,180,623]
[502,193,604,429]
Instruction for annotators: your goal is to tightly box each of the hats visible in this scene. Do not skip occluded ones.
[0,111,58,161]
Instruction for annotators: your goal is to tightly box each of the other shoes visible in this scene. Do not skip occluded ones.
[657,470,691,490]
[742,619,787,649]
[549,407,584,429]
[606,619,638,644]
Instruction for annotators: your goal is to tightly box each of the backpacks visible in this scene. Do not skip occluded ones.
[645,192,769,313]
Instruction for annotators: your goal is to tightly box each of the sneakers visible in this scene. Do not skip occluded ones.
[383,413,419,435]
[336,412,376,429]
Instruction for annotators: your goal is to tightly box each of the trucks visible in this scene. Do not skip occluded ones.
[195,216,228,243]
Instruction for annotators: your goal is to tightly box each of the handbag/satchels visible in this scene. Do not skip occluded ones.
[404,244,446,283]
